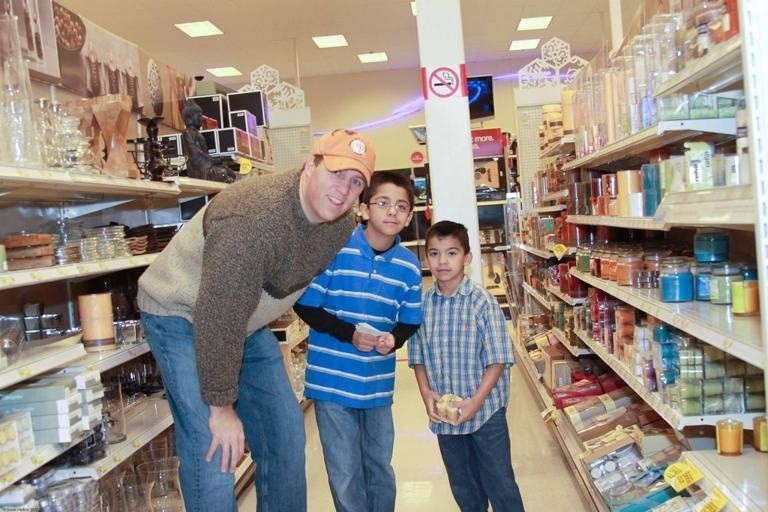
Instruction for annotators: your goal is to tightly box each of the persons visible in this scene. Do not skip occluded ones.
[408,220,525,512]
[292,173,423,512]
[136,127,377,512]
[181,99,240,184]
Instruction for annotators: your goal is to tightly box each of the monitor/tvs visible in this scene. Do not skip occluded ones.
[466,75,495,123]
[424,157,508,202]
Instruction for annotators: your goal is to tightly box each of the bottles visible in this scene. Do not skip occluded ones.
[0,14,188,512]
[520,14,767,511]
[286,355,315,406]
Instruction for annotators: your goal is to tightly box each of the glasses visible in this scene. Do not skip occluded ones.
[367,200,409,212]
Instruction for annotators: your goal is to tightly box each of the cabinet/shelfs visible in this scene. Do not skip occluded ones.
[1,106,315,512]
[358,127,518,326]
[509,1,767,512]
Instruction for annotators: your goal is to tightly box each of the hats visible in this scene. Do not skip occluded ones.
[311,128,374,188]
